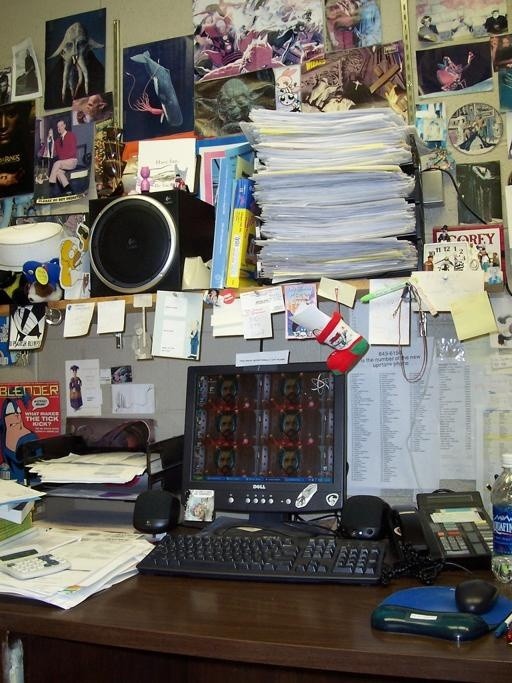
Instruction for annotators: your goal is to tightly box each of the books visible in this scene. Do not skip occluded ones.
[210,159,262,291]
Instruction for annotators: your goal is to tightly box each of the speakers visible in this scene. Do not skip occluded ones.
[339,495,392,539]
[133,490,180,534]
[88,189,215,298]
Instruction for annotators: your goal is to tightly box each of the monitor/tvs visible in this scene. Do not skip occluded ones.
[182,360,347,535]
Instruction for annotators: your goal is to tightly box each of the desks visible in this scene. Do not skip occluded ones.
[1,522,509,683]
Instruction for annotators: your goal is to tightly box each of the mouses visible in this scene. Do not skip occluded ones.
[454,578,499,615]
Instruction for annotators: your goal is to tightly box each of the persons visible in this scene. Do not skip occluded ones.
[418,15,439,41]
[274,446,302,477]
[211,375,243,409]
[275,409,302,443]
[477,246,499,272]
[439,225,450,243]
[70,364,83,412]
[205,444,243,475]
[484,10,508,33]
[0,76,12,104]
[47,118,79,197]
[274,373,308,405]
[23,48,38,89]
[0,102,36,197]
[209,412,241,441]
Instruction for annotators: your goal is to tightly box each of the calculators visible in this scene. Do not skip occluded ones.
[0,544,70,580]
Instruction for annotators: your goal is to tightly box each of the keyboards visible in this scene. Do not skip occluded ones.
[136,533,387,588]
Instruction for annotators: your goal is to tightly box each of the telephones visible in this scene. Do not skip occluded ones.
[386,489,493,569]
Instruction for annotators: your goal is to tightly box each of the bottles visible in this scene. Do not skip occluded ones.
[490,453,512,584]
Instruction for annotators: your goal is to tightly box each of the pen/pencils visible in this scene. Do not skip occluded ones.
[360,277,419,303]
[494,613,512,638]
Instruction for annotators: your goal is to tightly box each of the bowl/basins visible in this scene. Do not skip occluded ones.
[0,223,70,272]
